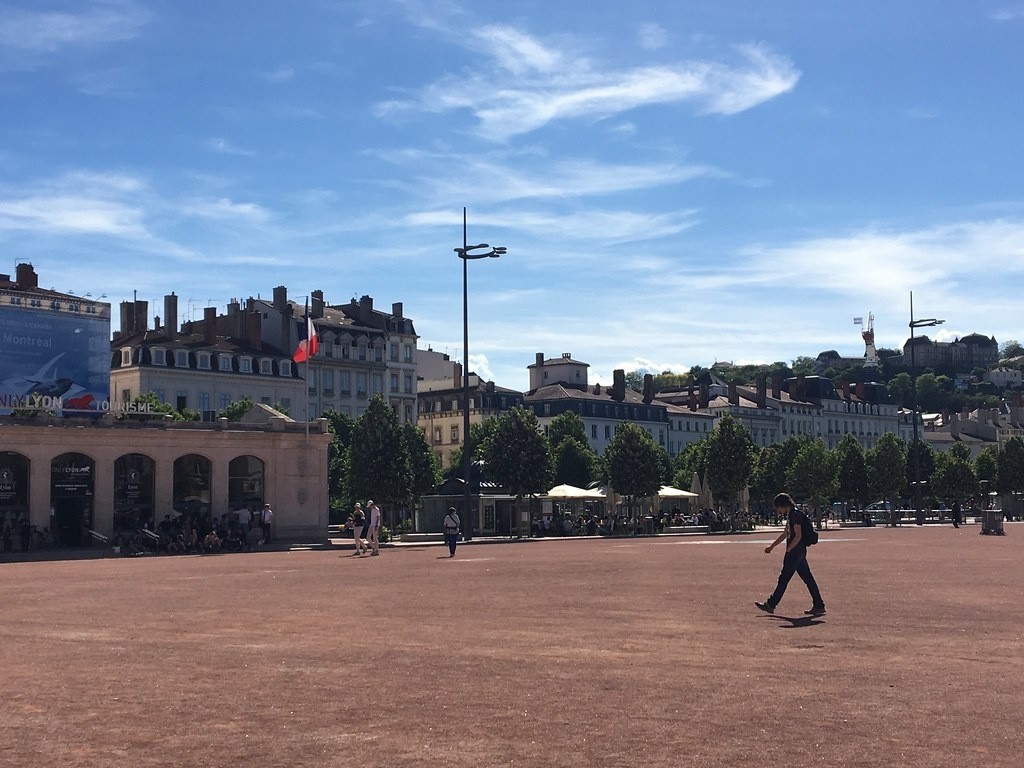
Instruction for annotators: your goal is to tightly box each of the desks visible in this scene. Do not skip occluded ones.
[509,527,528,537]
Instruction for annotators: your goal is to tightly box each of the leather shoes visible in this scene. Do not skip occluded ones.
[804,607,826,614]
[755,601,774,613]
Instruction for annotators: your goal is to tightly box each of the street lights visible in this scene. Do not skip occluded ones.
[909,318,946,524]
[454,243,507,541]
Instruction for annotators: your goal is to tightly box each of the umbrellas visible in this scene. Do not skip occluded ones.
[531,483,699,518]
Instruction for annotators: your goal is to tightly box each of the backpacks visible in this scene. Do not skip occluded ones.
[788,508,818,547]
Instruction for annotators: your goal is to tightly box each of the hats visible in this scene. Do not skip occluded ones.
[449,507,456,513]
[355,503,361,508]
[366,500,374,507]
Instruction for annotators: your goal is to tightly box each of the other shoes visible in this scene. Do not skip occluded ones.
[449,553,455,557]
[353,551,360,555]
[362,546,367,554]
[370,550,379,556]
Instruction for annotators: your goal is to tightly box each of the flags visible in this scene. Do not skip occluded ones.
[293,298,319,363]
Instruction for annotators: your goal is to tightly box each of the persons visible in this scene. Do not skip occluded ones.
[3,530,13,554]
[261,504,272,544]
[939,500,997,528]
[114,504,255,554]
[345,517,354,539]
[443,507,460,557]
[533,505,718,536]
[755,493,826,614]
[366,500,381,556]
[18,512,31,552]
[351,503,368,555]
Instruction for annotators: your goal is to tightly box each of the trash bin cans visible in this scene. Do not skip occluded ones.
[982,508,1004,531]
[644,516,653,532]
